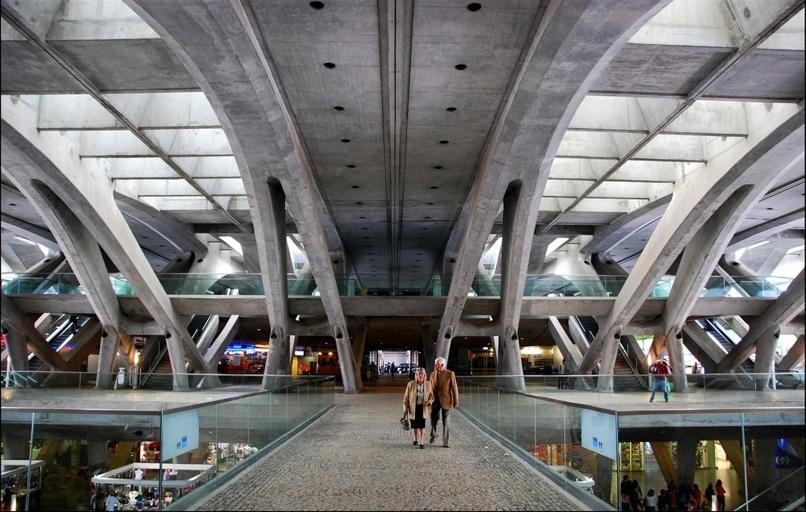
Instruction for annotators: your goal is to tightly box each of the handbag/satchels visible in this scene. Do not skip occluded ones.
[400,418,409,431]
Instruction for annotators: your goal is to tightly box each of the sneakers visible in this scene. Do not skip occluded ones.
[413,434,449,449]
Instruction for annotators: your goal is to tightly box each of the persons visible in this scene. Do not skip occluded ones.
[429,356,460,447]
[163,468,178,480]
[402,366,435,449]
[240,351,266,374]
[692,361,698,374]
[618,473,727,511]
[104,487,131,511]
[134,469,144,480]
[648,354,672,402]
[558,357,568,390]
[369,360,396,378]
